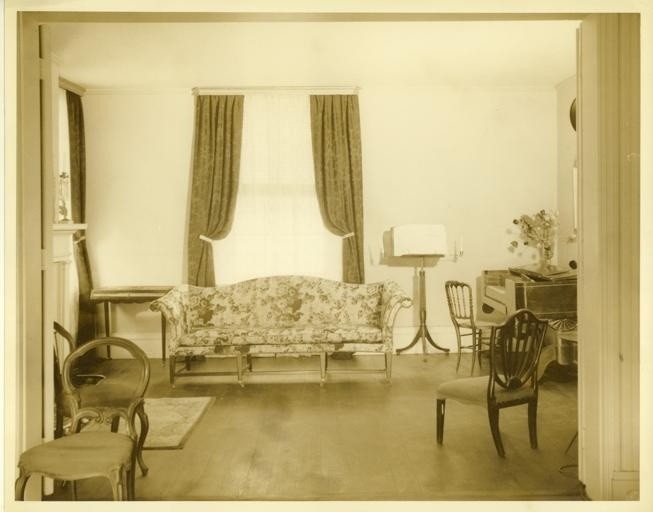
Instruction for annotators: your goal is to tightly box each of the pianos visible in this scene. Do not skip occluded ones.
[483,268,577,366]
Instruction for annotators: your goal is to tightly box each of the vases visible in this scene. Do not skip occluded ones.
[543,247,553,264]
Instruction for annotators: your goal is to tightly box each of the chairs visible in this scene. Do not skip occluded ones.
[54,321,149,477]
[445,280,497,375]
[15,337,151,501]
[437,309,548,458]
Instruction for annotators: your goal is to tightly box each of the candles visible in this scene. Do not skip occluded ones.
[460,234,463,251]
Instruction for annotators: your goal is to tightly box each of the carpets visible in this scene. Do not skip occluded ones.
[80,396,216,449]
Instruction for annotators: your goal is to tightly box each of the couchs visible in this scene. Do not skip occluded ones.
[150,275,413,388]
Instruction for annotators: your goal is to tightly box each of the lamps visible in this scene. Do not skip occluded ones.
[392,224,450,362]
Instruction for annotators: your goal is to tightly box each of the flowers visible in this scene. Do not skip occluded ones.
[510,209,558,250]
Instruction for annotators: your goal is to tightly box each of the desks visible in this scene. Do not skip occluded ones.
[90,286,172,361]
